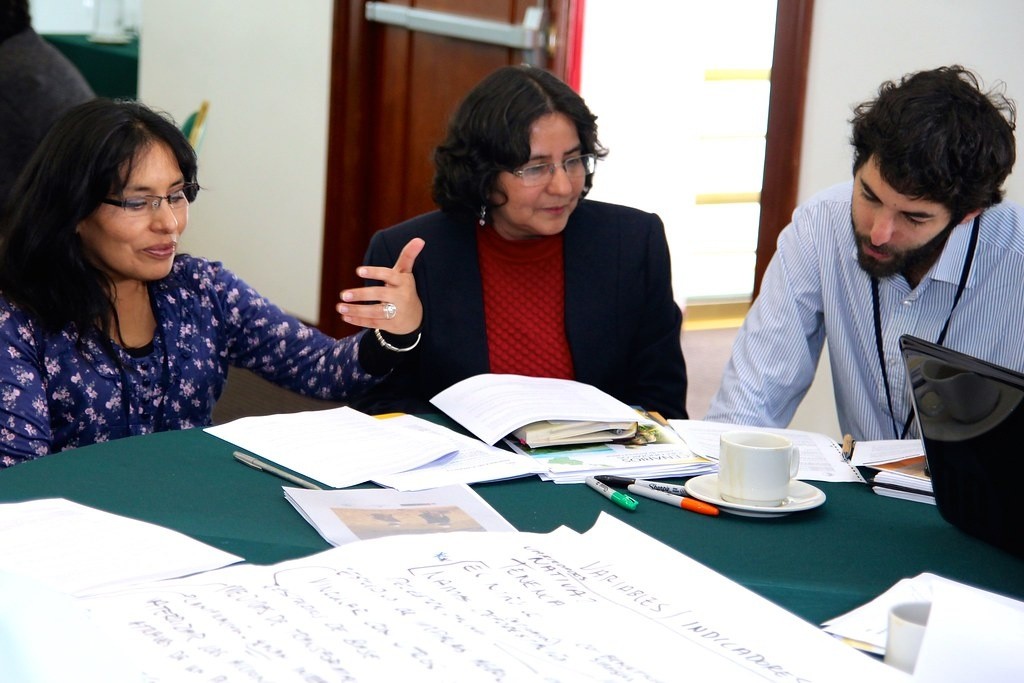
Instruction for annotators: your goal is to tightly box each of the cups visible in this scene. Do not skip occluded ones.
[718,430,799,507]
[883,600,931,675]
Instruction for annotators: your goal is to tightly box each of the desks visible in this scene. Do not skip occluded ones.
[0,409,1024,683]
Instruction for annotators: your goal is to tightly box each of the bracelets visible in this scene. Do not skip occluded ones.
[374,329,421,352]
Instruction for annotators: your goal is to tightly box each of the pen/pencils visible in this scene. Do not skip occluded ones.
[627,484,720,517]
[842,434,855,461]
[610,429,622,435]
[231,450,321,490]
[586,477,639,511]
[594,475,691,497]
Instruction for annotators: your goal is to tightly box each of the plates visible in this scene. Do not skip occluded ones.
[684,473,826,518]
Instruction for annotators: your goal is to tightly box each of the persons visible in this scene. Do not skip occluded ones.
[0,0,96,159]
[0,100,425,469]
[701,64,1024,440]
[359,62,689,419]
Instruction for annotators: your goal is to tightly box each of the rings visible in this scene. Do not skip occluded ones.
[383,302,396,320]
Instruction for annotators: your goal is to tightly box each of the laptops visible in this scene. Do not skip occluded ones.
[898,333,1024,561]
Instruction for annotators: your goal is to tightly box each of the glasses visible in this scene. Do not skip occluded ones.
[100,183,197,217]
[505,152,596,186]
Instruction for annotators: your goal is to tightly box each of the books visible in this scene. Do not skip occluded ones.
[502,405,717,485]
